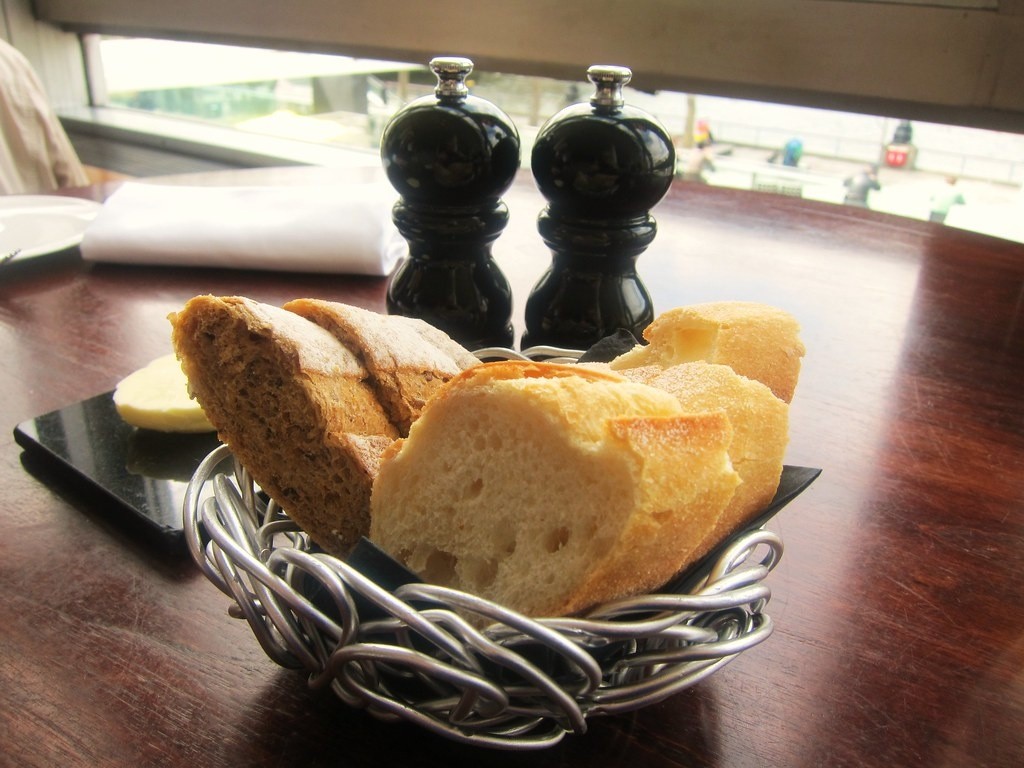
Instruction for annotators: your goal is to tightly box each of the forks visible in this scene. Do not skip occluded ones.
[1,250,23,265]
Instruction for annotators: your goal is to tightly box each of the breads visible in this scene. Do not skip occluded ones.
[110,294,803,629]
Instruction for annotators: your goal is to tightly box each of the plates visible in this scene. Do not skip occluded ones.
[1,195,102,266]
[14,387,268,536]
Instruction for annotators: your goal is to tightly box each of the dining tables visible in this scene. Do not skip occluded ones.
[1,170,1024,768]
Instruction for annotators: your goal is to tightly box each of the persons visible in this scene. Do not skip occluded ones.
[842,163,881,209]
[927,172,966,224]
[0,39,90,196]
[691,121,722,176]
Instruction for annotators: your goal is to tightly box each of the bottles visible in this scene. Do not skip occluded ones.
[520,64,675,353]
[381,57,520,350]
